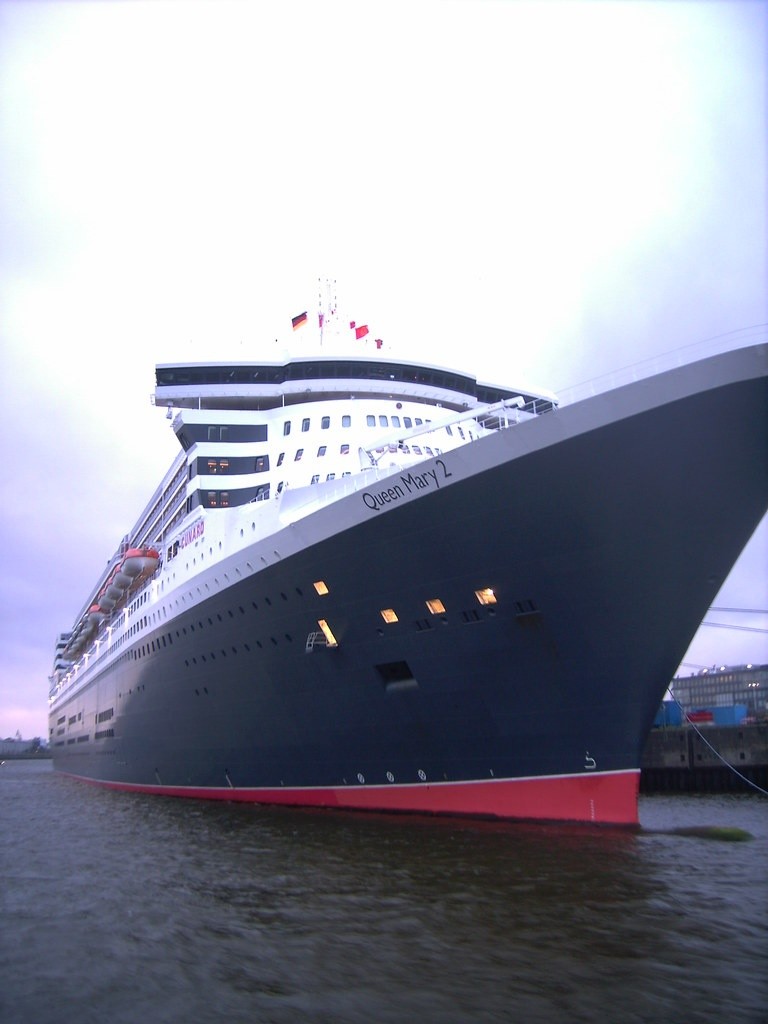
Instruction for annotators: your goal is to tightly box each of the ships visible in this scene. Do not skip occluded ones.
[48,275,767,830]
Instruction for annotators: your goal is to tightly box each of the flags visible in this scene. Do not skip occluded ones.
[319,315,324,328]
[356,325,369,339]
[292,311,309,332]
[350,321,355,329]
